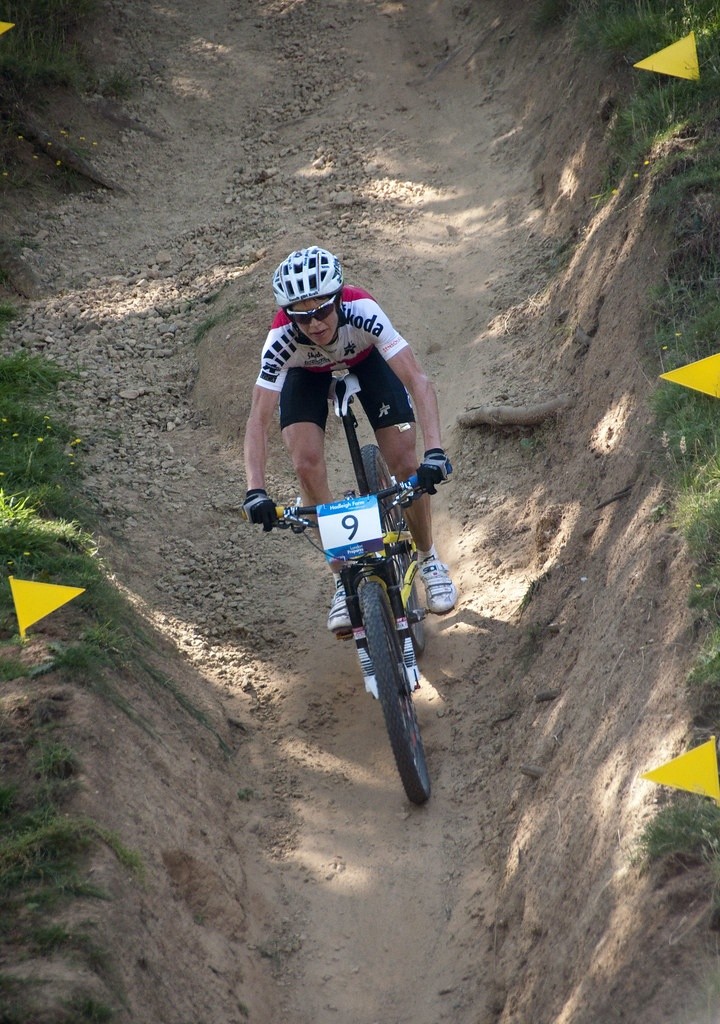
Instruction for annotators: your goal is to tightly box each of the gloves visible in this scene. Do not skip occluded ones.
[415,448,451,496]
[240,489,280,533]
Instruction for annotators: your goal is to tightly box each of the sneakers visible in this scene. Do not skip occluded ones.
[326,588,366,632]
[419,560,459,615]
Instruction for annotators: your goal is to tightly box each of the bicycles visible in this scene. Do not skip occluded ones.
[240,372,452,807]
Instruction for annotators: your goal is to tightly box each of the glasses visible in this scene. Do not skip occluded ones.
[286,294,337,325]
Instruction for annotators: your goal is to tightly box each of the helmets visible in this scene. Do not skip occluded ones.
[270,245,344,307]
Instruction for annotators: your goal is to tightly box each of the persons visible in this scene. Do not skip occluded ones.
[239,245,457,631]
